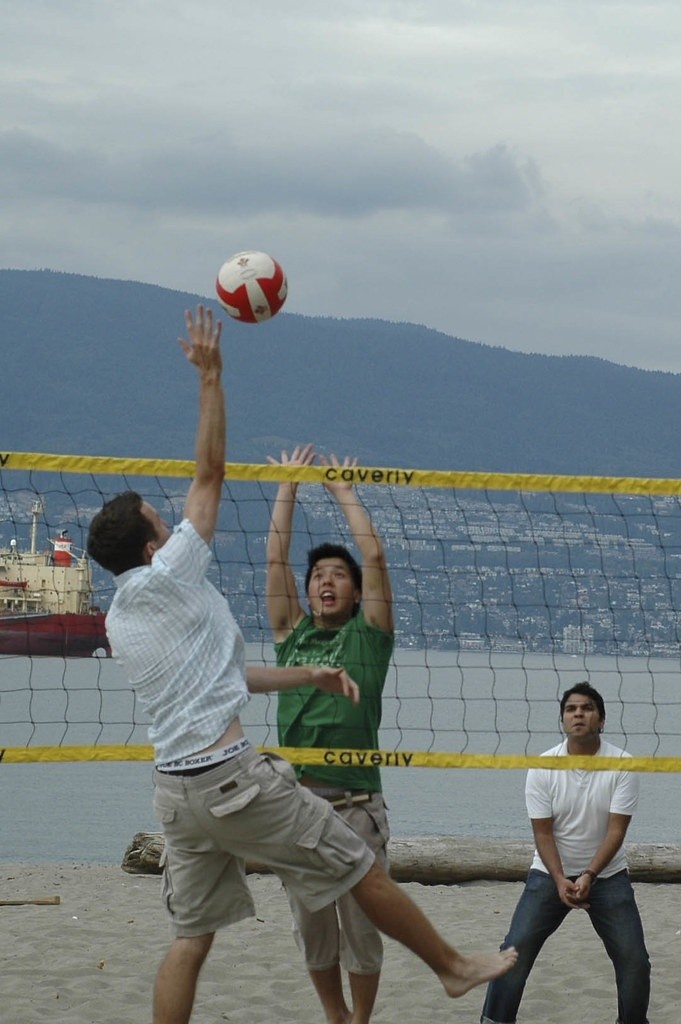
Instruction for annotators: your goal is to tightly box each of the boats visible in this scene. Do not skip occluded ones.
[0,497,112,658]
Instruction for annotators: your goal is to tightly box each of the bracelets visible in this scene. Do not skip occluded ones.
[579,870,597,886]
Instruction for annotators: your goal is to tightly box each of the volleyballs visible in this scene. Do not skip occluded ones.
[217,252,292,324]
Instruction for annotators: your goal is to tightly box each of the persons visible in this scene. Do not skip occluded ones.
[260,443,397,1024]
[482,682,651,1024]
[90,305,512,1024]
